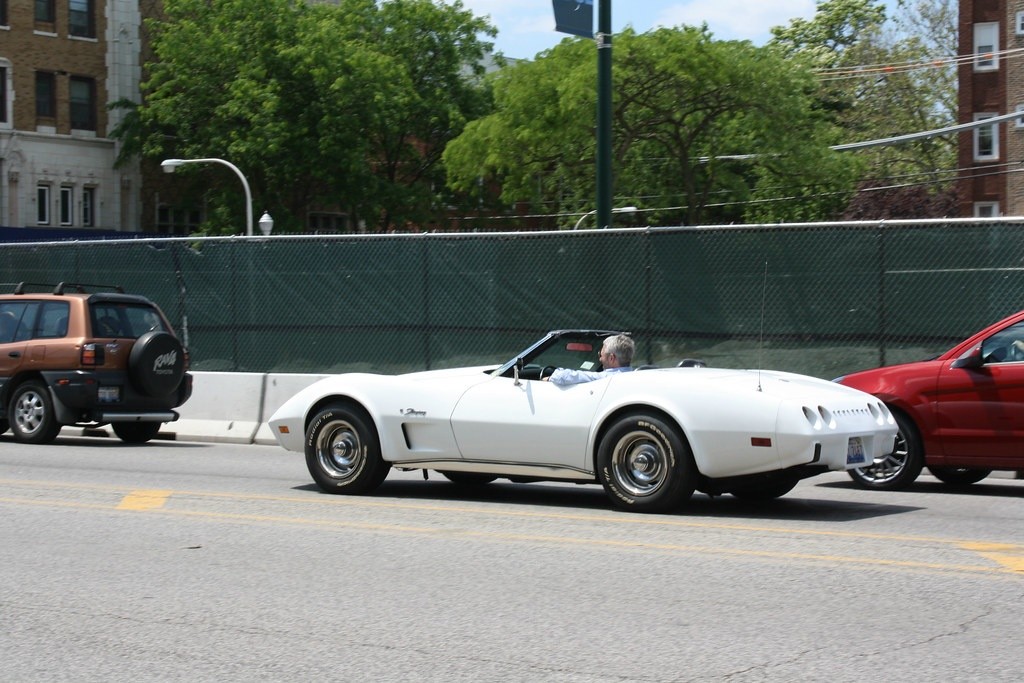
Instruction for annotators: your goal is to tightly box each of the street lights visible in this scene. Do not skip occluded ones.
[160,157,253,237]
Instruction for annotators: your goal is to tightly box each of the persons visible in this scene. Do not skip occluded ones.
[540,334,635,386]
[1012,339,1024,352]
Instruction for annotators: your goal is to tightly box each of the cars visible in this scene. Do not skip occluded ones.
[828,310,1023,491]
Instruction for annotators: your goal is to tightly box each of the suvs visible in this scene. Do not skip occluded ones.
[2,279,194,442]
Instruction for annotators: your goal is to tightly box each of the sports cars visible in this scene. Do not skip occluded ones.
[266,261,900,514]
